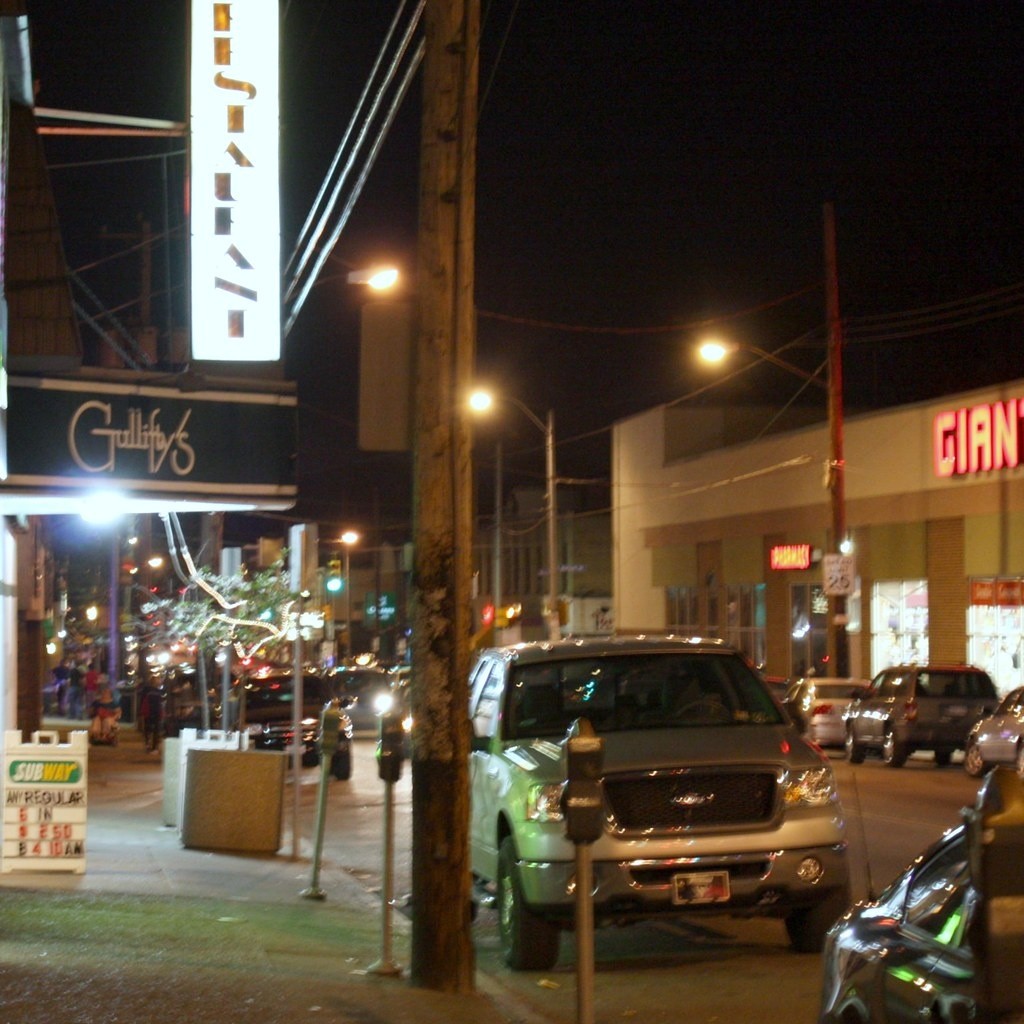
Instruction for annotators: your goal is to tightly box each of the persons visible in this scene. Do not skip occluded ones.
[51,658,102,718]
[141,686,164,751]
[88,688,121,736]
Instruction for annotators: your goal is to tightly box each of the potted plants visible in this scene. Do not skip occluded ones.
[129,540,320,855]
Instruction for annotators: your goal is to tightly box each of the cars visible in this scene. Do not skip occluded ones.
[821,768,1024,1024]
[129,628,398,771]
[782,671,871,751]
[841,663,999,770]
[963,682,1024,779]
[463,628,848,974]
